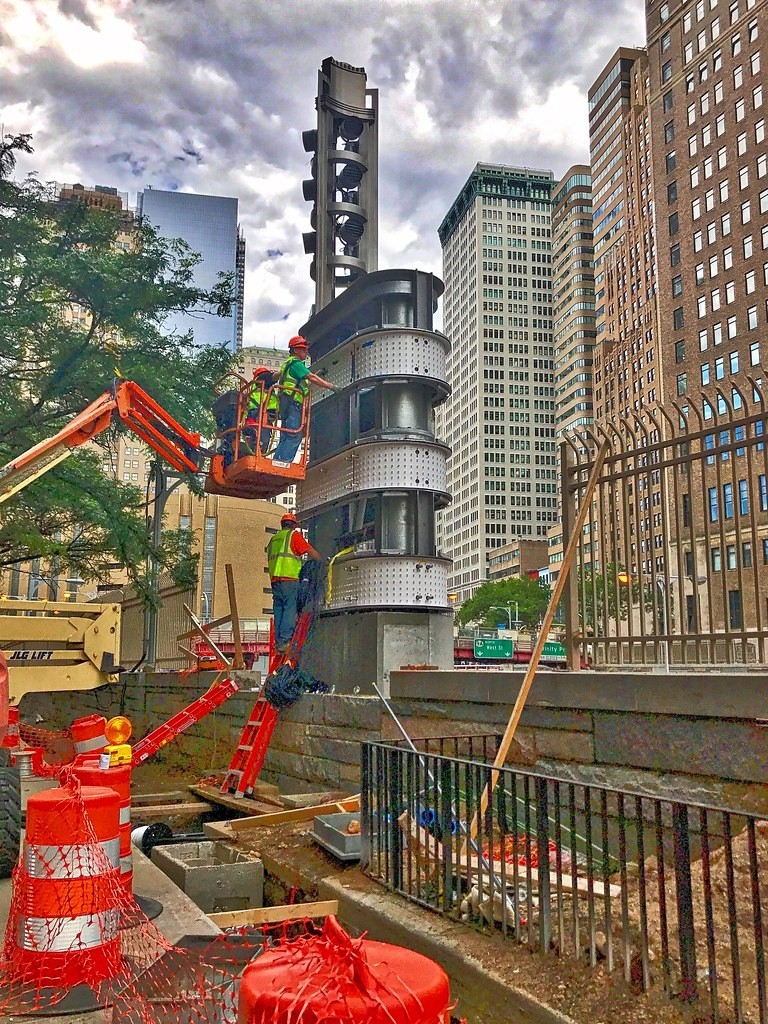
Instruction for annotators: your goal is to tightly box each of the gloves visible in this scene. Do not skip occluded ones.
[330,385,343,394]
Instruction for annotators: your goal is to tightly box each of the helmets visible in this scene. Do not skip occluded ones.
[252,368,271,380]
[288,335,310,348]
[280,513,298,524]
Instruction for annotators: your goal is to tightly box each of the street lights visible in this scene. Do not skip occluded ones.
[618,572,707,673]
[201,591,209,625]
[490,607,511,630]
[507,600,523,641]
[26,573,85,616]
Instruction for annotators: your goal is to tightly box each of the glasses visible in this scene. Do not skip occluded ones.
[304,341,310,345]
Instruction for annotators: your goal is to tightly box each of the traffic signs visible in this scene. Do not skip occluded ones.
[474,638,513,659]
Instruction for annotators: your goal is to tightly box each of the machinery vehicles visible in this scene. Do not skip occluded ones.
[0,366,312,880]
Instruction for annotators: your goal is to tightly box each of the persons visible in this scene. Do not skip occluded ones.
[273,336,343,462]
[267,513,322,656]
[242,367,281,457]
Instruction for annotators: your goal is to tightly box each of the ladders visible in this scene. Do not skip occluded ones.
[131,677,241,770]
[219,612,311,799]
